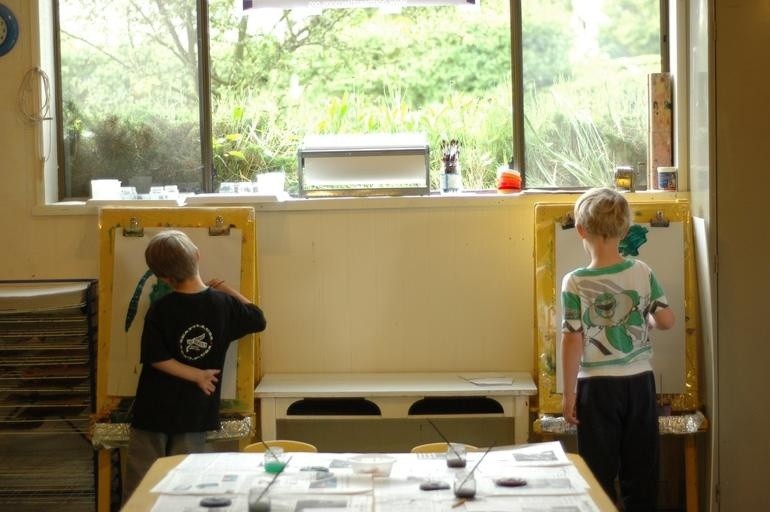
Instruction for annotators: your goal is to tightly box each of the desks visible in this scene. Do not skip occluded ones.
[117,452,618,512]
[245,371,537,445]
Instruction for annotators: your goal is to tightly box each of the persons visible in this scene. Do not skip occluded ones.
[558,185,677,507]
[115,229,267,512]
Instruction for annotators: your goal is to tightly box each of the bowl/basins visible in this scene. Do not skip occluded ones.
[348,453,396,477]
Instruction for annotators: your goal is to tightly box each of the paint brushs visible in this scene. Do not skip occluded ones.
[660,375,663,409]
[426,417,463,464]
[456,440,497,492]
[244,421,279,462]
[254,456,293,504]
[214,280,225,289]
[125,398,136,418]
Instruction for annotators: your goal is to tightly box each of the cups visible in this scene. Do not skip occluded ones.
[454,471,478,498]
[90,177,179,201]
[615,171,635,193]
[439,161,463,195]
[446,443,467,468]
[657,166,677,190]
[220,172,284,195]
[264,446,284,475]
[246,487,271,512]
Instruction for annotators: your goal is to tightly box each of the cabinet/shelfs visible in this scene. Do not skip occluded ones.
[0,279,98,512]
